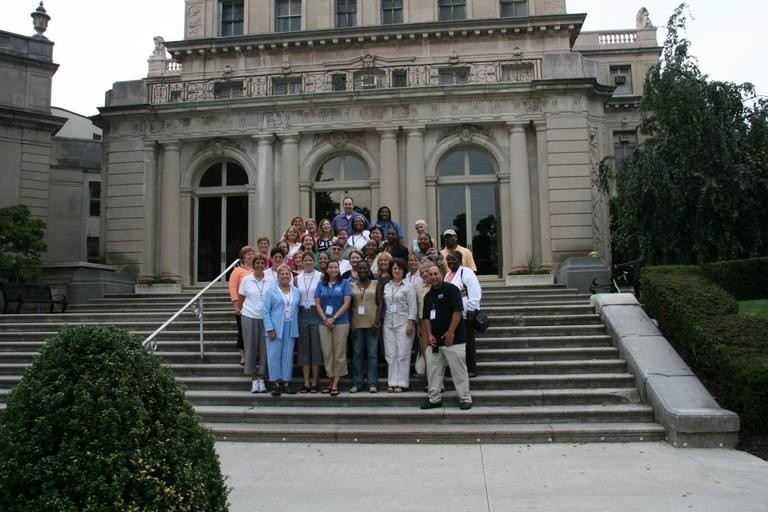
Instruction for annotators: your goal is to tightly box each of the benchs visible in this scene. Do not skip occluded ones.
[2,283,68,313]
[588,258,642,298]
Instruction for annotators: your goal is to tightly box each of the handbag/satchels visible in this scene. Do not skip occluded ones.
[414,354,426,375]
[472,310,489,333]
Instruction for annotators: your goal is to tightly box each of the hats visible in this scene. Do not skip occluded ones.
[443,229,456,236]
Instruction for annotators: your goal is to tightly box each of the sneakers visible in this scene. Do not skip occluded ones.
[252,379,402,397]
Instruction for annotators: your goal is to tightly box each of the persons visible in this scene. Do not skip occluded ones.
[228,194,483,411]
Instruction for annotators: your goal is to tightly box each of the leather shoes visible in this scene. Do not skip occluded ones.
[460,402,472,410]
[421,401,442,409]
